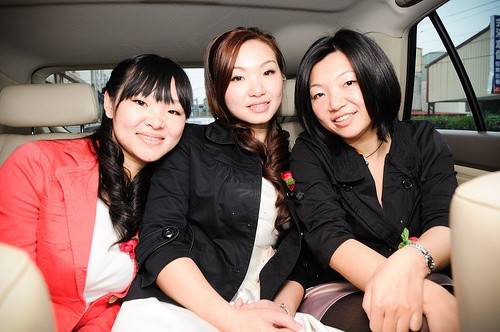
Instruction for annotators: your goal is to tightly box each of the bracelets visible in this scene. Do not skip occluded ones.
[279,303,289,315]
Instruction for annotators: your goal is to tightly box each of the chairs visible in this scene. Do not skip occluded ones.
[0,76,500,332]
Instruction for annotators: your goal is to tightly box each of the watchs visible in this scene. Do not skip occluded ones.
[403,242,436,275]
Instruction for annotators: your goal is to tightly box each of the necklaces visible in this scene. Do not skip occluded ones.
[364,141,383,165]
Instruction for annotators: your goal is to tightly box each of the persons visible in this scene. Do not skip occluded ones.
[290,28,460,332]
[0,54,193,332]
[111,27,345,332]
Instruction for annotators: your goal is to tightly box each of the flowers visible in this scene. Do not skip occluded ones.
[121,232,140,258]
[398,227,419,249]
[281,170,296,192]
